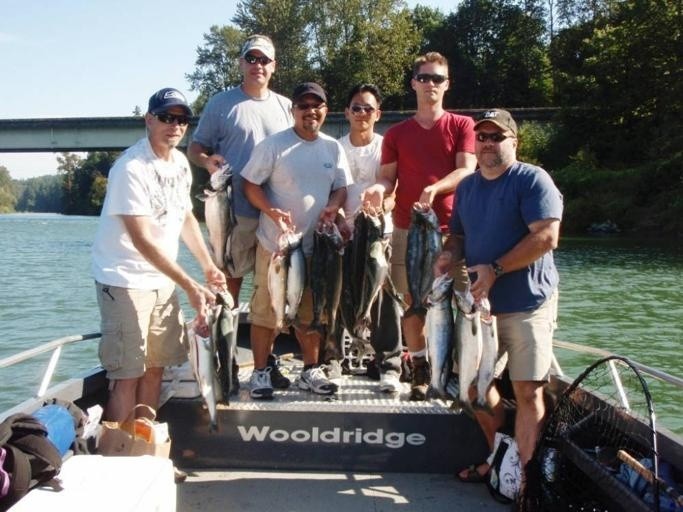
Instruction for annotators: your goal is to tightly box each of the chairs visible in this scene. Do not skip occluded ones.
[175,466,188,480]
[246,352,432,400]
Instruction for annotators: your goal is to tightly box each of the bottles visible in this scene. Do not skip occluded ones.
[149,88,193,119]
[241,34,276,61]
[293,82,327,104]
[474,108,517,137]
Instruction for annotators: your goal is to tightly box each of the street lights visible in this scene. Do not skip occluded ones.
[484,432,521,503]
[96,403,171,459]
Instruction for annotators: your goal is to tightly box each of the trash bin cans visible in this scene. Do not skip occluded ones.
[491,261,504,278]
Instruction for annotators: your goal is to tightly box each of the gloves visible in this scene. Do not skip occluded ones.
[0,297,683,512]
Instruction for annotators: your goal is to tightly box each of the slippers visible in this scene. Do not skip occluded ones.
[0,413,62,512]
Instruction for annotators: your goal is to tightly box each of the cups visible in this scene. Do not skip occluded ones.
[292,102,328,110]
[150,111,191,126]
[476,132,516,142]
[414,74,448,84]
[352,104,380,113]
[245,54,271,65]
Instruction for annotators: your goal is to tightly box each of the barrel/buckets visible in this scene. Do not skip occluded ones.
[458,464,485,482]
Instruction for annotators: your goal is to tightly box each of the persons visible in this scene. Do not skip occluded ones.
[318,83,404,393]
[186,34,296,399]
[432,107,564,512]
[359,52,478,400]
[90,88,228,422]
[239,83,356,399]
